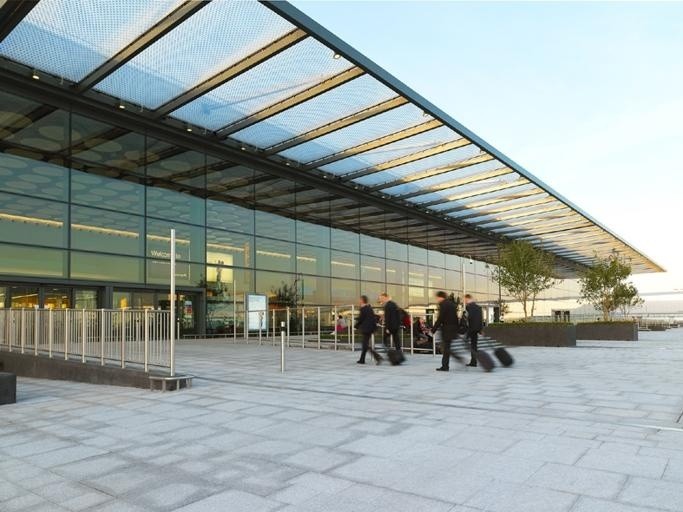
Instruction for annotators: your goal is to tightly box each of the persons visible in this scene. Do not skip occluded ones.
[337,315,346,331]
[379,293,401,351]
[354,295,384,365]
[499,314,504,322]
[431,292,462,370]
[462,311,468,328]
[413,317,429,338]
[463,294,484,367]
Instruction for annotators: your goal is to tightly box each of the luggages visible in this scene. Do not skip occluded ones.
[388,350,404,365]
[495,347,512,366]
[476,350,493,369]
